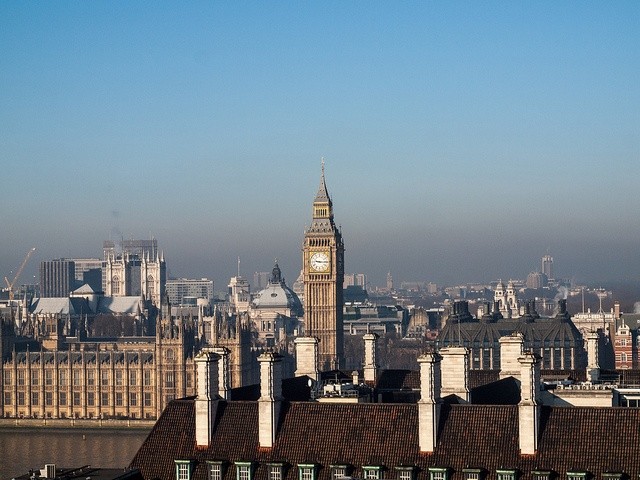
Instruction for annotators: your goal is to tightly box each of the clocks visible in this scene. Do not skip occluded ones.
[309,251,329,273]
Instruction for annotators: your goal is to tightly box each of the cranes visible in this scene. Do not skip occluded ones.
[4,248,36,299]
